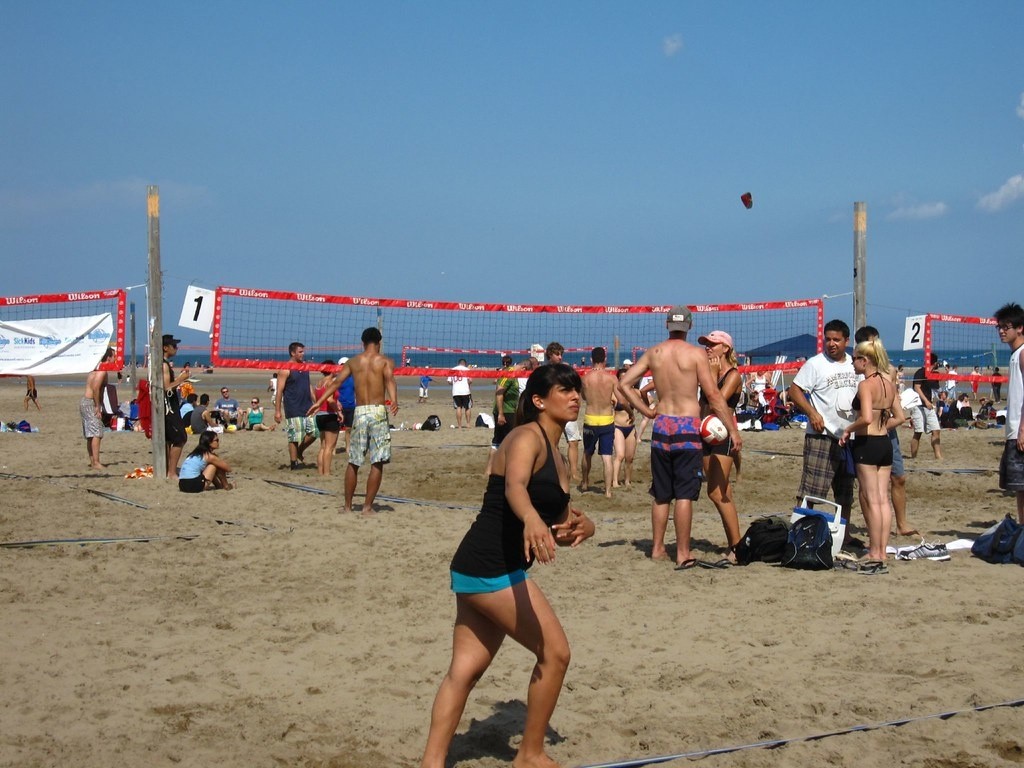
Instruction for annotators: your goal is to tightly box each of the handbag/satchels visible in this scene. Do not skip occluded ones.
[971,511,1024,566]
[421,414,441,430]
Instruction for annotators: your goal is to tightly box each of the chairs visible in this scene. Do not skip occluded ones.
[750,386,793,429]
[125,398,139,431]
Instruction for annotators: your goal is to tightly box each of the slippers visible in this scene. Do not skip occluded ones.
[674,559,699,570]
[699,558,732,570]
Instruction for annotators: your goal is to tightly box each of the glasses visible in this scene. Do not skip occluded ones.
[251,402,258,404]
[214,439,218,442]
[995,324,1014,331]
[851,355,863,361]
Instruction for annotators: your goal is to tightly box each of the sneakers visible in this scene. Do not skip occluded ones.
[900,537,951,561]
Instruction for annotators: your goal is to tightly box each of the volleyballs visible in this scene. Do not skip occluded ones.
[700,415,728,444]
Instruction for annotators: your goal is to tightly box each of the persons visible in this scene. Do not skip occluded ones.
[447,359,473,429]
[855,326,917,536]
[169,359,281,434]
[698,331,741,561]
[24,376,41,410]
[993,303,1024,525]
[406,357,410,366]
[163,335,191,479]
[418,376,437,403]
[80,348,115,469]
[838,339,906,561]
[117,363,130,384]
[735,369,794,430]
[789,319,858,544]
[306,327,398,514]
[617,307,743,570]
[178,431,236,493]
[488,341,655,497]
[275,342,355,475]
[420,364,595,768]
[896,353,1003,458]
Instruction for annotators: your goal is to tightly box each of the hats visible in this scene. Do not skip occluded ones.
[623,359,633,365]
[698,331,732,349]
[666,306,692,332]
[162,334,181,345]
[338,357,348,365]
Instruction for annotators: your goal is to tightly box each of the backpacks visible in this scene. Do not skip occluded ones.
[732,511,835,571]
